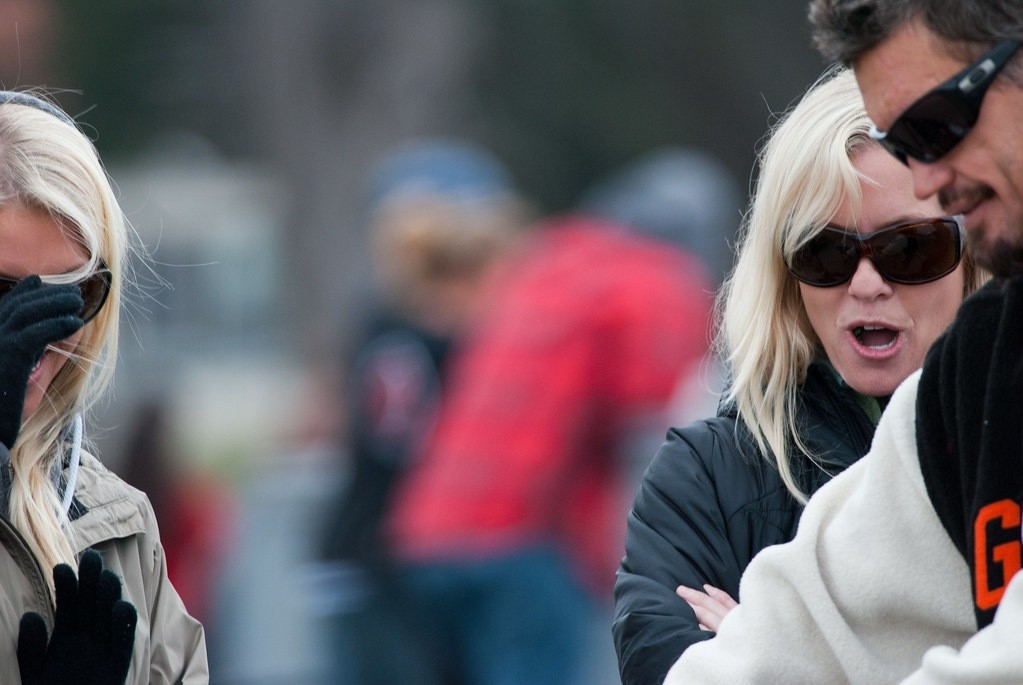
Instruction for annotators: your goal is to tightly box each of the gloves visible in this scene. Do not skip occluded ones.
[17,550,137,685]
[1,275,85,450]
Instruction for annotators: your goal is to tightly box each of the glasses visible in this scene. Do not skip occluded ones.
[1,258,113,325]
[868,37,1023,167]
[783,214,966,288]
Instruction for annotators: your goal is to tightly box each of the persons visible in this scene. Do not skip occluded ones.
[609,65,993,685]
[117,144,738,685]
[0,86,210,685]
[664,1,1023,685]
